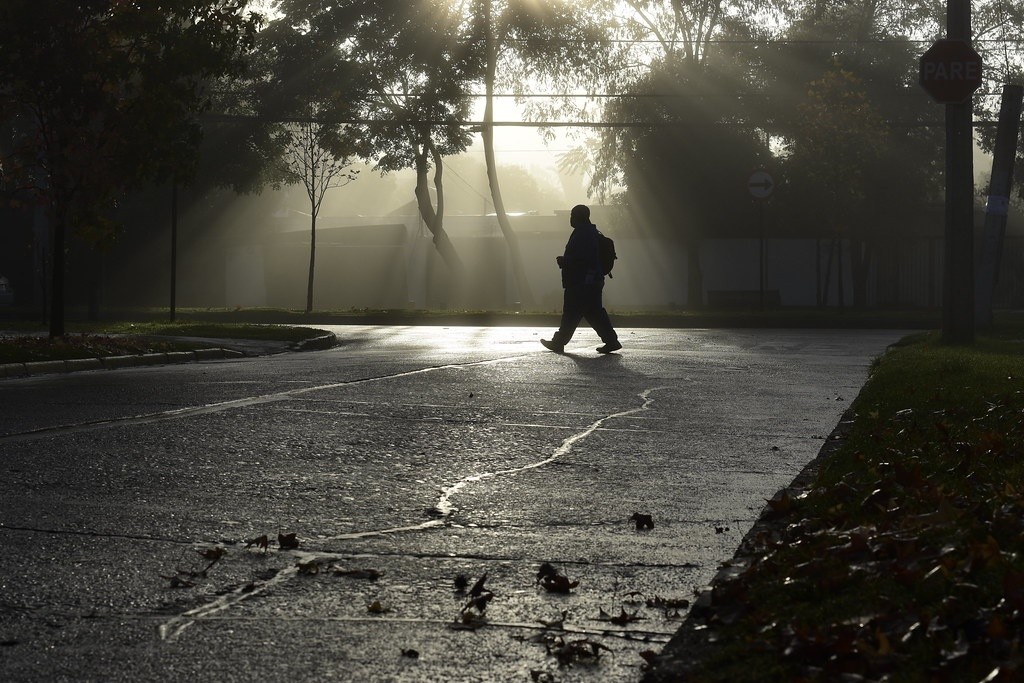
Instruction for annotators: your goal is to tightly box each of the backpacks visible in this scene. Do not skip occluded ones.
[596,229,618,279]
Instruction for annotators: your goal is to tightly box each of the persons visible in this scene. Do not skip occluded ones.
[540,205,622,355]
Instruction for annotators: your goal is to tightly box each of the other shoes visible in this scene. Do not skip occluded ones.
[596,341,622,352]
[540,338,564,354]
